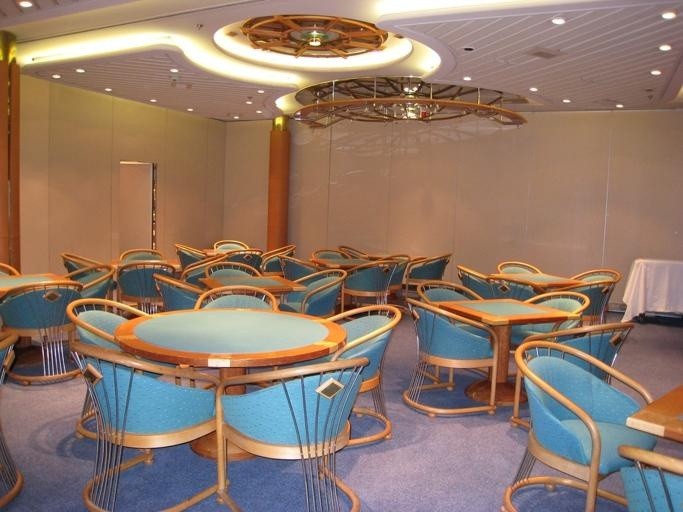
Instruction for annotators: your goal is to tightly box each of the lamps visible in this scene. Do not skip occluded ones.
[368,76,441,119]
